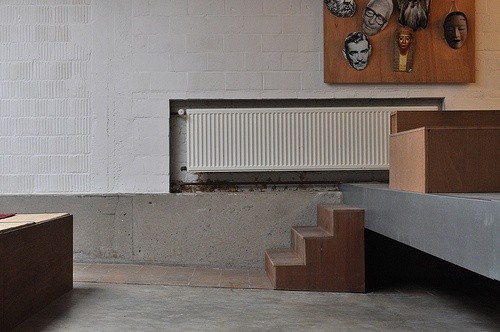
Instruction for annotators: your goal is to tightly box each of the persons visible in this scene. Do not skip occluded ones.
[442,11,469,49]
[392,27,415,72]
[394,0,431,34]
[361,0,394,36]
[341,31,372,71]
[325,0,357,19]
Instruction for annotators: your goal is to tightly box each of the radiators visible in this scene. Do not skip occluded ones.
[177,105,436,174]
